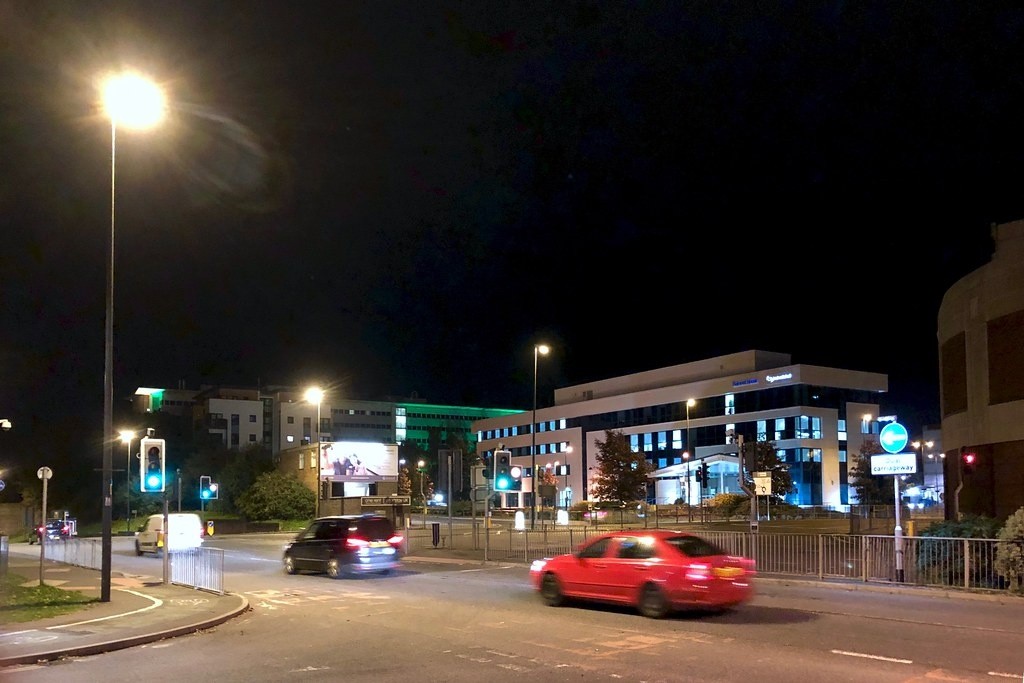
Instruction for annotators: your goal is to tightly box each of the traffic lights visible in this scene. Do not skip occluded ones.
[140,439,165,492]
[959,446,973,475]
[696,470,701,482]
[493,450,523,493]
[199,475,218,499]
[482,447,497,480]
[703,462,710,488]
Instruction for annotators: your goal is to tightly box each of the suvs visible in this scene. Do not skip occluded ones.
[283,513,404,580]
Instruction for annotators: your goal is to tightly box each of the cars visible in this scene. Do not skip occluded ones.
[529,527,757,620]
[28,519,71,546]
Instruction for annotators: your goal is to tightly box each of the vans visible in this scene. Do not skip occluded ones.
[135,512,205,557]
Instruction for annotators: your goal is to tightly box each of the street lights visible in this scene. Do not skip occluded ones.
[686,398,696,524]
[529,344,549,532]
[120,430,135,531]
[305,386,323,517]
